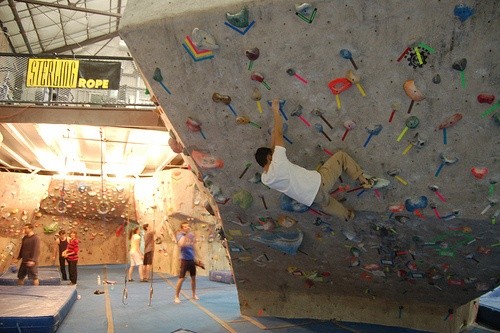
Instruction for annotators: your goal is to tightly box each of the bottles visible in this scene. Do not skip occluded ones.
[96,275,101,286]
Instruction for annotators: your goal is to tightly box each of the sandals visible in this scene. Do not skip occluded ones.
[361,177,377,191]
[345,210,355,224]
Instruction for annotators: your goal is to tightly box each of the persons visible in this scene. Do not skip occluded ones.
[141,220,157,281]
[52,229,72,285]
[62,231,79,285]
[173,222,206,303]
[127,225,148,282]
[13,223,41,286]
[254,99,390,223]
